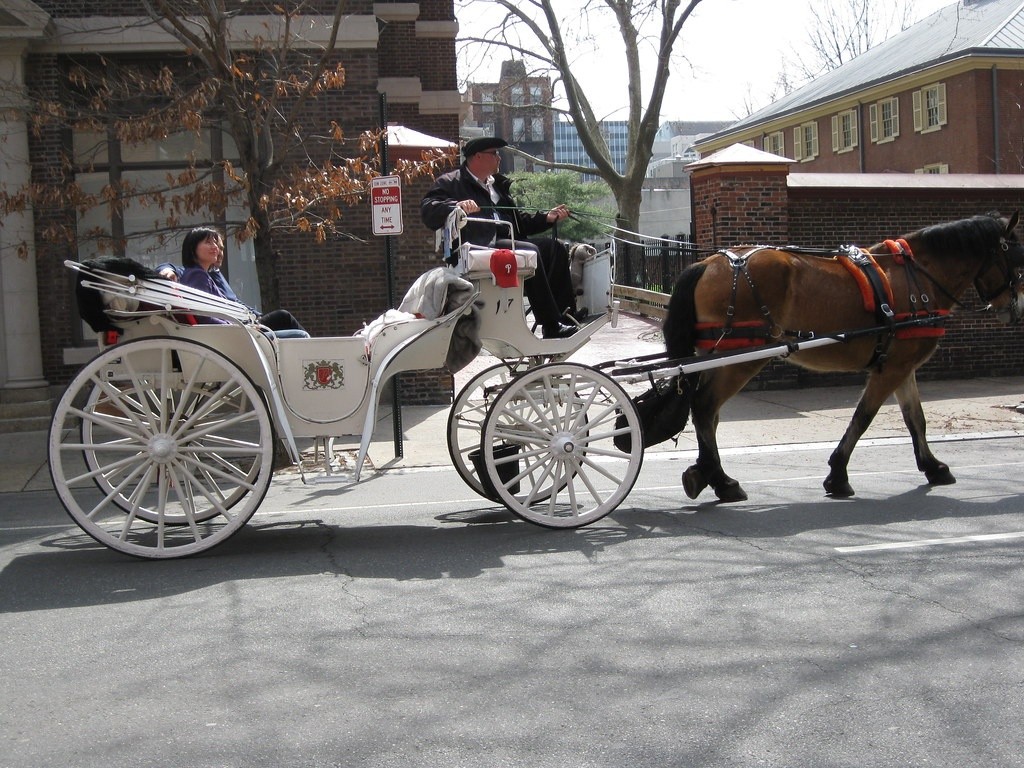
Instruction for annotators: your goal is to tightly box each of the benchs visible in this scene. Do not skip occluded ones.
[449,248,537,277]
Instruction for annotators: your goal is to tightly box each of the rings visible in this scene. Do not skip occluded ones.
[469,200,474,203]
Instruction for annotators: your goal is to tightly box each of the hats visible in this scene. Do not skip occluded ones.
[491,249,520,289]
[463,137,508,157]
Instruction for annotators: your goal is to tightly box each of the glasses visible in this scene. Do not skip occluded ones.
[473,151,500,157]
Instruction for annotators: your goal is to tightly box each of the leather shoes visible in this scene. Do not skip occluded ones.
[542,322,580,339]
[562,307,589,325]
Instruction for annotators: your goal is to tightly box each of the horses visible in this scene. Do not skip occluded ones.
[664,211,1024,503]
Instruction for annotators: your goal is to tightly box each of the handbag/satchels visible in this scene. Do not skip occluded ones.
[614,374,691,453]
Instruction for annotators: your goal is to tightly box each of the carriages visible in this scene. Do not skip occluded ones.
[46,196,1024,561]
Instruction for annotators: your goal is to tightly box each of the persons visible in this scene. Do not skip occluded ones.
[422,136,587,339]
[155,227,308,338]
